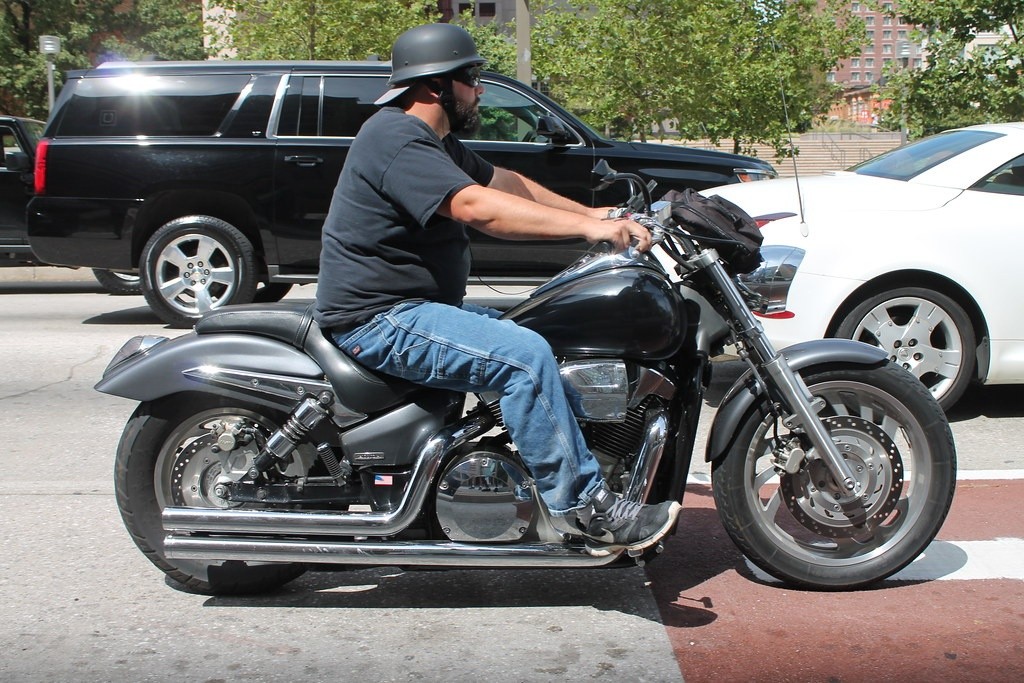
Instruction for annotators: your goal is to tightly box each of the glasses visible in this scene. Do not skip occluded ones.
[453,66,481,88]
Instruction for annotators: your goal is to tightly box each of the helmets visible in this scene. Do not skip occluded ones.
[386,23,487,88]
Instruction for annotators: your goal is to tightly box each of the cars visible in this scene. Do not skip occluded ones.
[628,122,1024,420]
[0,115,147,295]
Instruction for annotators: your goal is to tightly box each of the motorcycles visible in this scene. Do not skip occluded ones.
[91,159,959,597]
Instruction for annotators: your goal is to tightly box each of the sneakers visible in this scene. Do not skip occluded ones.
[575,486,682,557]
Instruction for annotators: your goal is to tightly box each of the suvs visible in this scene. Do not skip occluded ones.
[22,56,780,332]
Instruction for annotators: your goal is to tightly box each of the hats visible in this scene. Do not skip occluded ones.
[373,79,418,105]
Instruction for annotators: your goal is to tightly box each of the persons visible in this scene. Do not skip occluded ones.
[313,22,686,556]
[871,113,878,133]
